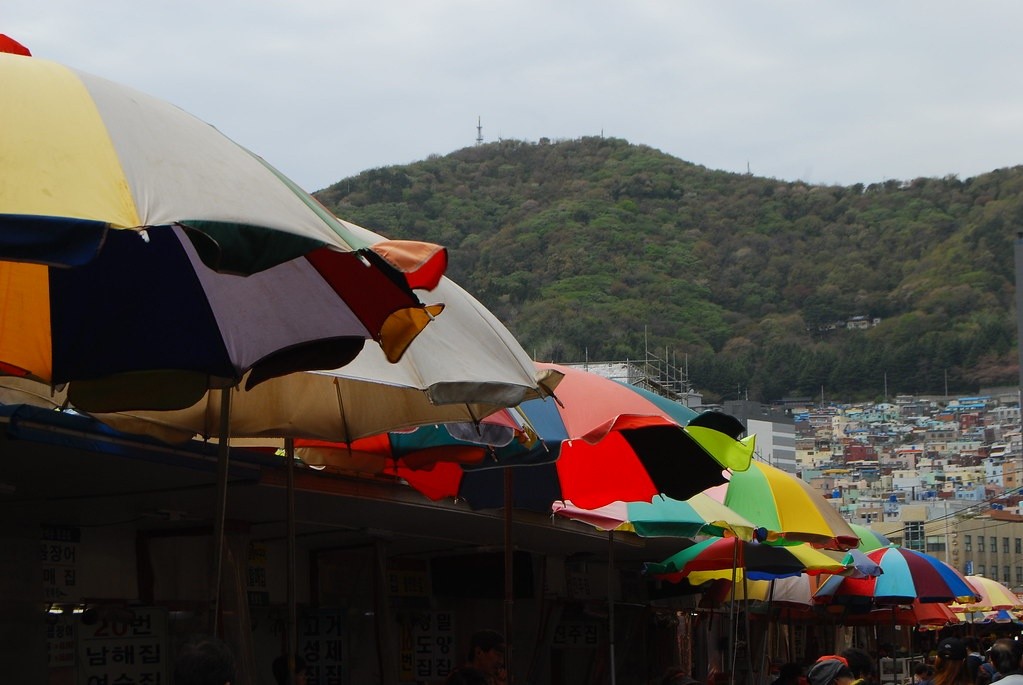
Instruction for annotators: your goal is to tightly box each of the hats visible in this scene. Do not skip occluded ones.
[937,638,967,660]
[807,659,845,685]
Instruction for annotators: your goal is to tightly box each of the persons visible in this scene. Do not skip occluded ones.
[444,632,515,685]
[662,666,703,685]
[174,638,236,685]
[772,628,1023,685]
[807,637,819,663]
[272,655,308,685]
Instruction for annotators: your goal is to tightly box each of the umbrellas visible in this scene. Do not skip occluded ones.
[912,576,1023,635]
[641,535,884,685]
[698,522,983,685]
[1,34,756,685]
[551,460,860,685]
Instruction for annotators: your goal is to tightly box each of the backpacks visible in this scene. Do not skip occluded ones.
[983,663,1002,683]
